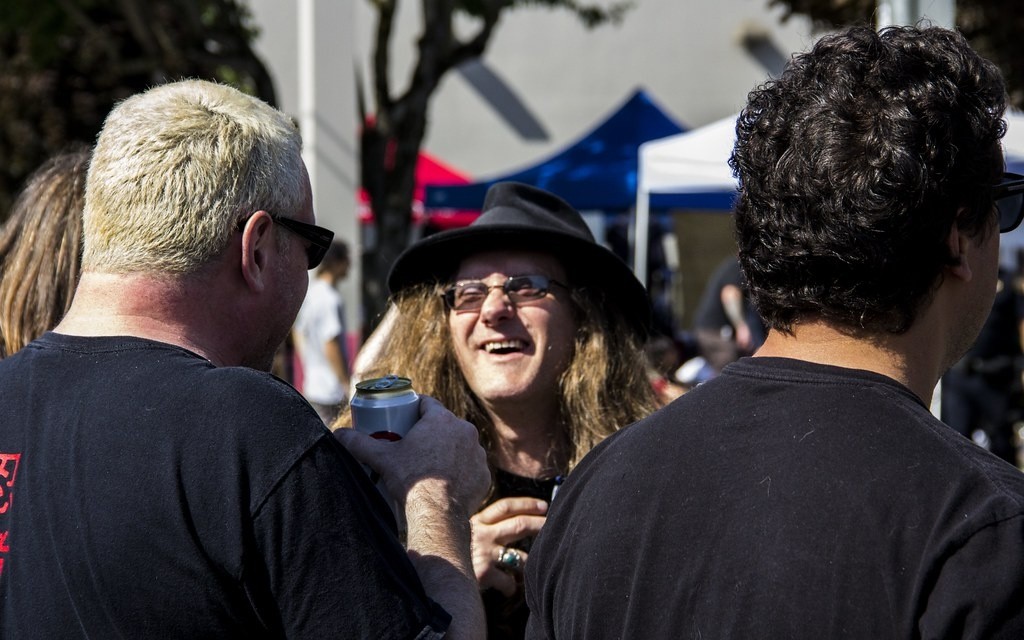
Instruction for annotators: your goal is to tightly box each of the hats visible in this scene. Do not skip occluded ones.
[387,181,653,343]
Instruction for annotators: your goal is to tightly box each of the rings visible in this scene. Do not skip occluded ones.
[497,547,520,572]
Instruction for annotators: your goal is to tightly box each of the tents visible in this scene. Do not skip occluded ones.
[422,86,741,214]
[631,96,1024,293]
[354,112,483,249]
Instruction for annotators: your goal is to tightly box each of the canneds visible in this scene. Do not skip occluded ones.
[350,376,420,548]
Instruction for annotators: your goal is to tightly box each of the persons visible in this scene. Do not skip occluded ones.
[327,179,661,640]
[0,144,92,357]
[0,80,495,640]
[520,21,1024,640]
[692,247,753,373]
[287,237,352,426]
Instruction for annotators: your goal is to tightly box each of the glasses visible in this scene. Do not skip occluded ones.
[444,275,583,311]
[979,172,1024,233]
[237,213,334,270]
